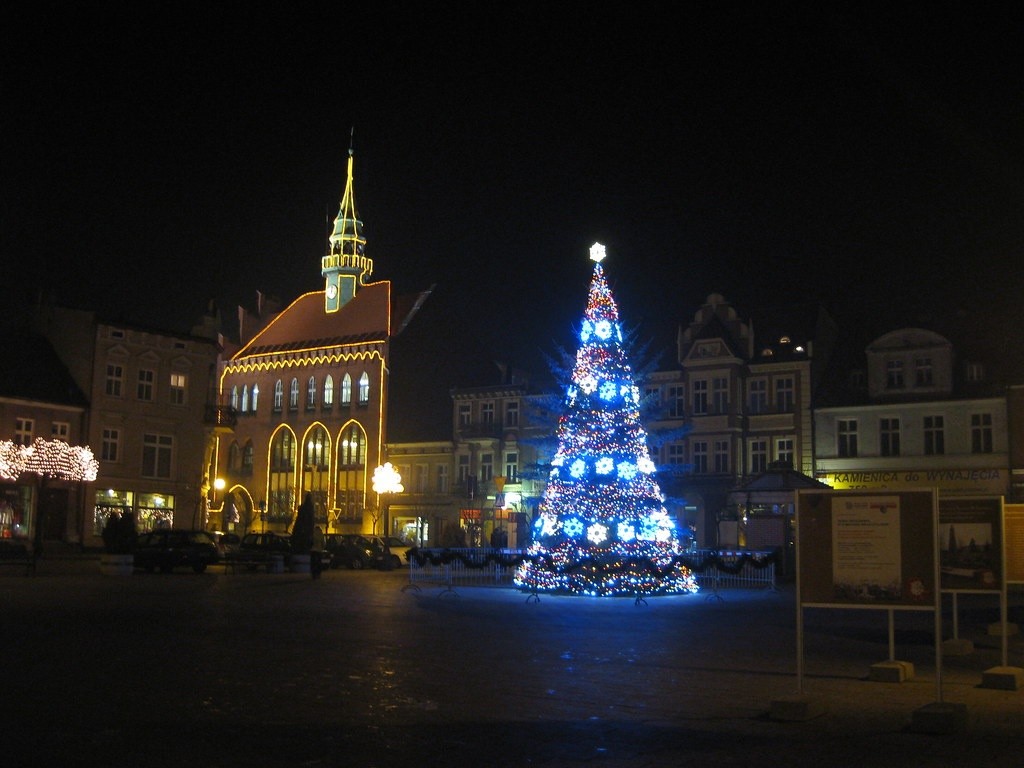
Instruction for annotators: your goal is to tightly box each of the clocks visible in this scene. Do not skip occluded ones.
[327,284,338,299]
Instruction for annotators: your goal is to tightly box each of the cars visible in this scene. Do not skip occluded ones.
[132,527,220,574]
[239,529,413,571]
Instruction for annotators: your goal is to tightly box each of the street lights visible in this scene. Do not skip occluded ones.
[371,462,404,571]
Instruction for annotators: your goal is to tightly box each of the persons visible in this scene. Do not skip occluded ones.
[310,526,324,581]
